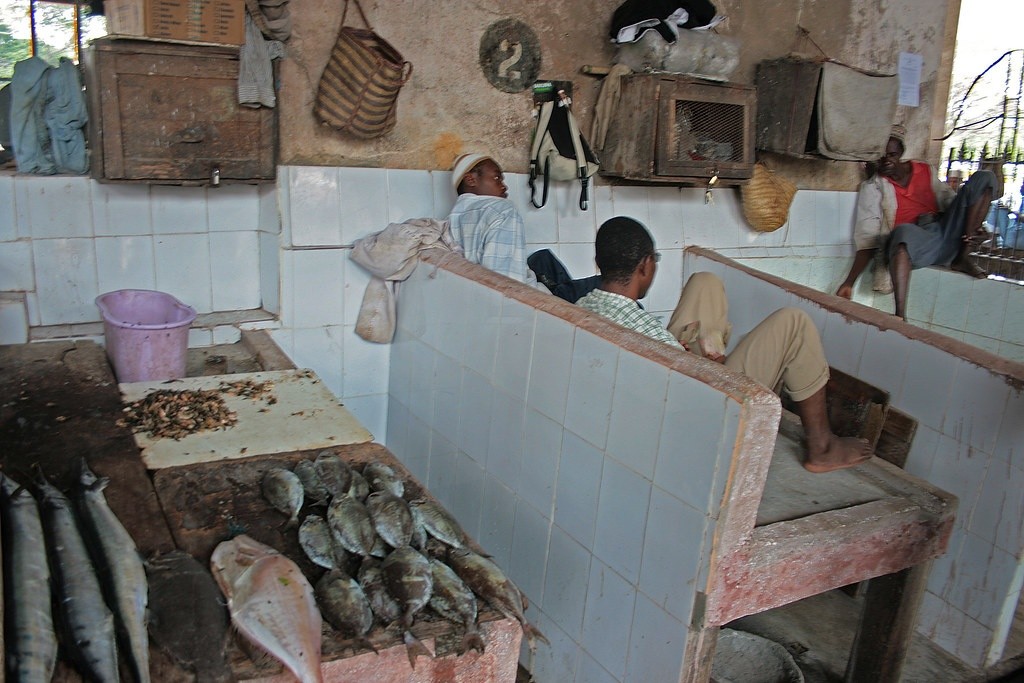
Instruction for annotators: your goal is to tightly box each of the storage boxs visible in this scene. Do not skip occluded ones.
[104,1,245,46]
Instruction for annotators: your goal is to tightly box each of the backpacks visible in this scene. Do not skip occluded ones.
[528,100,600,212]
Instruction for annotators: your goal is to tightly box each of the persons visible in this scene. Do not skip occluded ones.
[836,122,1000,324]
[437,154,645,315]
[945,169,963,192]
[575,217,875,473]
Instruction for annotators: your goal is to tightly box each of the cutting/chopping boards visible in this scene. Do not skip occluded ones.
[116,369,376,472]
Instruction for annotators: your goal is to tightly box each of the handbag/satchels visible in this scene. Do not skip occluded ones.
[743,162,798,234]
[313,0,414,139]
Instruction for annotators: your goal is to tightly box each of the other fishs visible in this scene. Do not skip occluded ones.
[0,457,151,683]
[261,450,551,670]
[142,533,323,683]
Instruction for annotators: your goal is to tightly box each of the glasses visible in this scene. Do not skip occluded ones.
[651,252,663,262]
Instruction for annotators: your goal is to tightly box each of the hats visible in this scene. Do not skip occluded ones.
[948,170,964,179]
[889,124,908,140]
[452,154,490,188]
